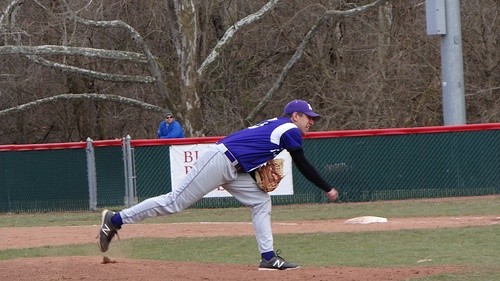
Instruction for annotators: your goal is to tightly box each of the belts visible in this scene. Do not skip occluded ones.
[225,151,242,174]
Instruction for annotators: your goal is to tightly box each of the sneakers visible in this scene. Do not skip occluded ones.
[258,249,299,270]
[99,209,122,252]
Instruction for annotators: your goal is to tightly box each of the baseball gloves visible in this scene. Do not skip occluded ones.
[255,158,282,193]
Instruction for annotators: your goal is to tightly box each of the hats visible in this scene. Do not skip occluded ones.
[284,100,321,122]
[162,112,173,118]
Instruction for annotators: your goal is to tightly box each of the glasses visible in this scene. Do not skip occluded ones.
[165,116,173,119]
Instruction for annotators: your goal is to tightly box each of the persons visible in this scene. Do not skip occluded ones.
[156,111,186,141]
[97,97,339,272]
